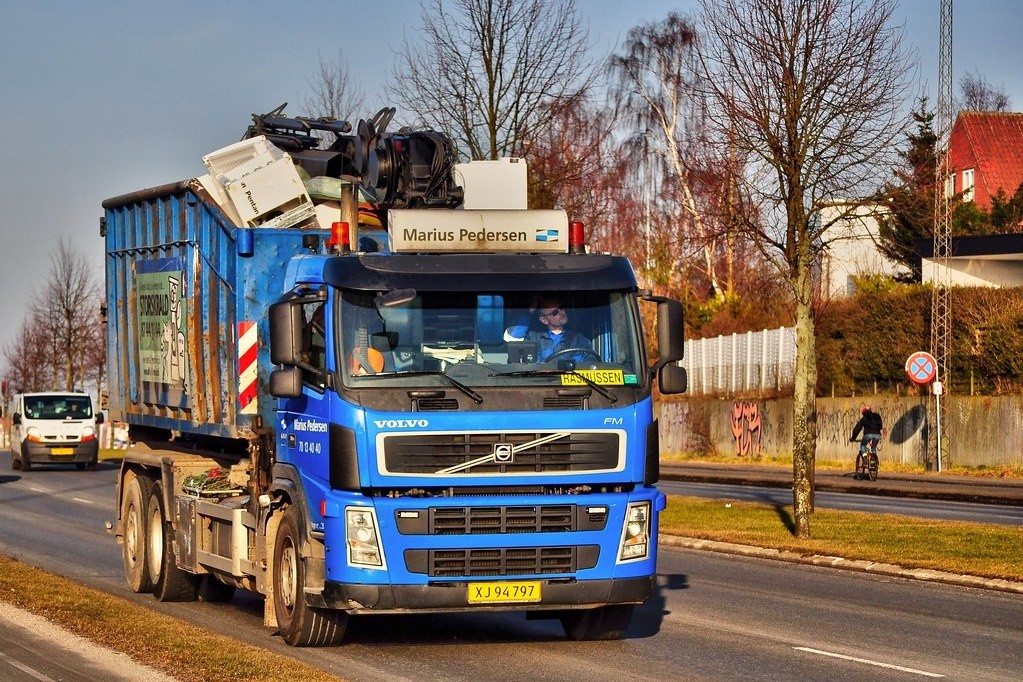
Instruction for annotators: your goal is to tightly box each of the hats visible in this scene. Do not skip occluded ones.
[861,407,870,412]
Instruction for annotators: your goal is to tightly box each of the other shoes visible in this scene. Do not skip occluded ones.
[862,461,868,467]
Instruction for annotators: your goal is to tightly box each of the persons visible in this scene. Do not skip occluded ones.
[850,406,883,467]
[502,300,598,363]
[65,404,87,416]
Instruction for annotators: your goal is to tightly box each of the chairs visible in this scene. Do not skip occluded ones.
[353,291,425,374]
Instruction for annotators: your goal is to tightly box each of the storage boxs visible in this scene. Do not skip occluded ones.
[218,145,317,230]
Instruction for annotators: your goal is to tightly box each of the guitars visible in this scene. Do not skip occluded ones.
[346,301,384,374]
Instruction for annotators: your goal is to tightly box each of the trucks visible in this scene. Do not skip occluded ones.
[9,389,105,471]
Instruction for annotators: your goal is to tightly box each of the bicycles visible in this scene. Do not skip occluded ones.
[850,437,880,481]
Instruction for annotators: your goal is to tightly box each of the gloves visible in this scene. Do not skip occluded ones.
[849,438,854,442]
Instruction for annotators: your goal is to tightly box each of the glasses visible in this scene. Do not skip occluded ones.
[538,306,565,317]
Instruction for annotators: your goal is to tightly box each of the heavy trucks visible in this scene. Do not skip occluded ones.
[94,96,690,652]
